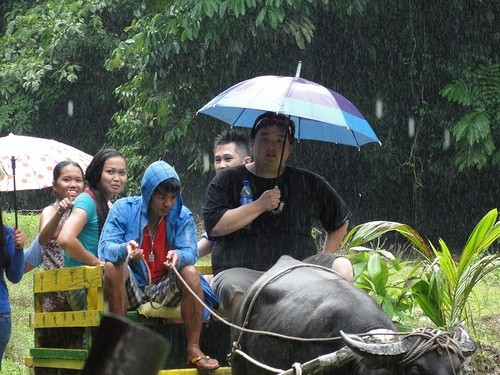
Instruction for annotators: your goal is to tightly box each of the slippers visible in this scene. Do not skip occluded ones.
[185,354,220,371]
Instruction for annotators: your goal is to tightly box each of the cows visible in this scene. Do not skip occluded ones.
[231,255,477,375]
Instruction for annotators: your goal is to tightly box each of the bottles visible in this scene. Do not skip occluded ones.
[238,181,253,230]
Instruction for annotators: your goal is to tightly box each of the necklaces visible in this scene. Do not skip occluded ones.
[148,216,164,262]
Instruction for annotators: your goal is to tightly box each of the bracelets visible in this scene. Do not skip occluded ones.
[57,209,64,216]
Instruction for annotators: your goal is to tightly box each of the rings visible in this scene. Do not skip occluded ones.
[19,238,21,240]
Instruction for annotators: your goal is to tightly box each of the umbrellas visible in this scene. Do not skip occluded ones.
[0,132,95,247]
[195,61,382,215]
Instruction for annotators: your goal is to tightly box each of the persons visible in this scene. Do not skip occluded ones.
[202,112,354,321]
[0,129,251,371]
